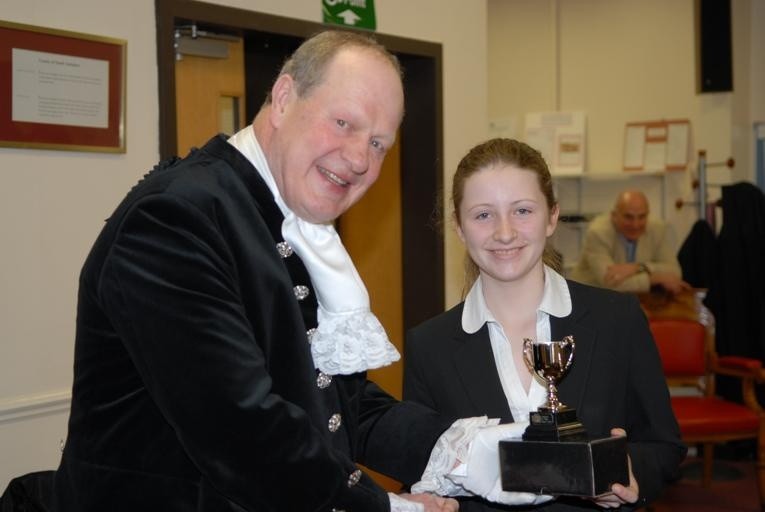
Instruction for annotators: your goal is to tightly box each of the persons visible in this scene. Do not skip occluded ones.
[675,181,765,460]
[571,189,695,295]
[401,135,686,511]
[46,29,554,511]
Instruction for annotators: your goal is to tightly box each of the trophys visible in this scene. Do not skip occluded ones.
[519,335,587,441]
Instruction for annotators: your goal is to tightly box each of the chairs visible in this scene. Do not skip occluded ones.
[645,313,764,491]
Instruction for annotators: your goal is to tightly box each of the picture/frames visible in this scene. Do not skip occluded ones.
[0,20,129,153]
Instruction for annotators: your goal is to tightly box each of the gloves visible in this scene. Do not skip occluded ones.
[446,419,553,505]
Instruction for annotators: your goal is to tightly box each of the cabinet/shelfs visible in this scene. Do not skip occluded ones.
[548,163,672,282]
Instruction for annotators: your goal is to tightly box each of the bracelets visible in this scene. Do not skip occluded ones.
[638,262,645,274]
